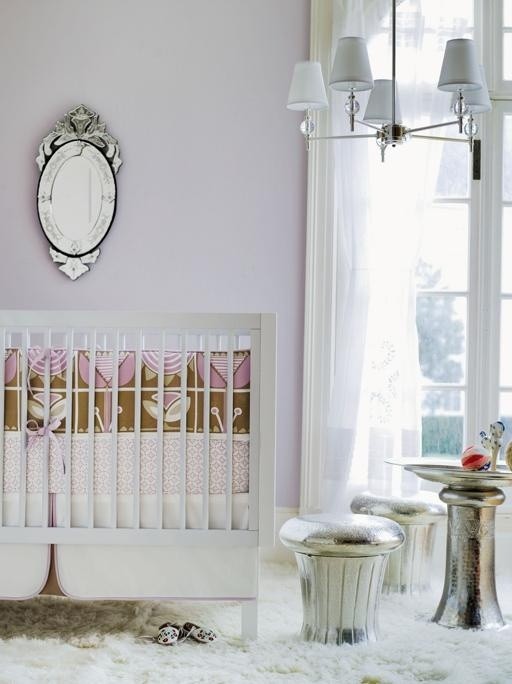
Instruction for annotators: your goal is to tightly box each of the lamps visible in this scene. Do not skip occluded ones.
[284,0,492,166]
[34,104,123,281]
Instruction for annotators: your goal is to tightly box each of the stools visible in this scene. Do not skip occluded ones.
[277,488,448,647]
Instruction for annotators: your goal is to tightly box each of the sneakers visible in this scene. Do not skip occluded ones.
[158,622,181,644]
[183,622,216,643]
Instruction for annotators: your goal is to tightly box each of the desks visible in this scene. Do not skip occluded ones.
[385,457,512,633]
